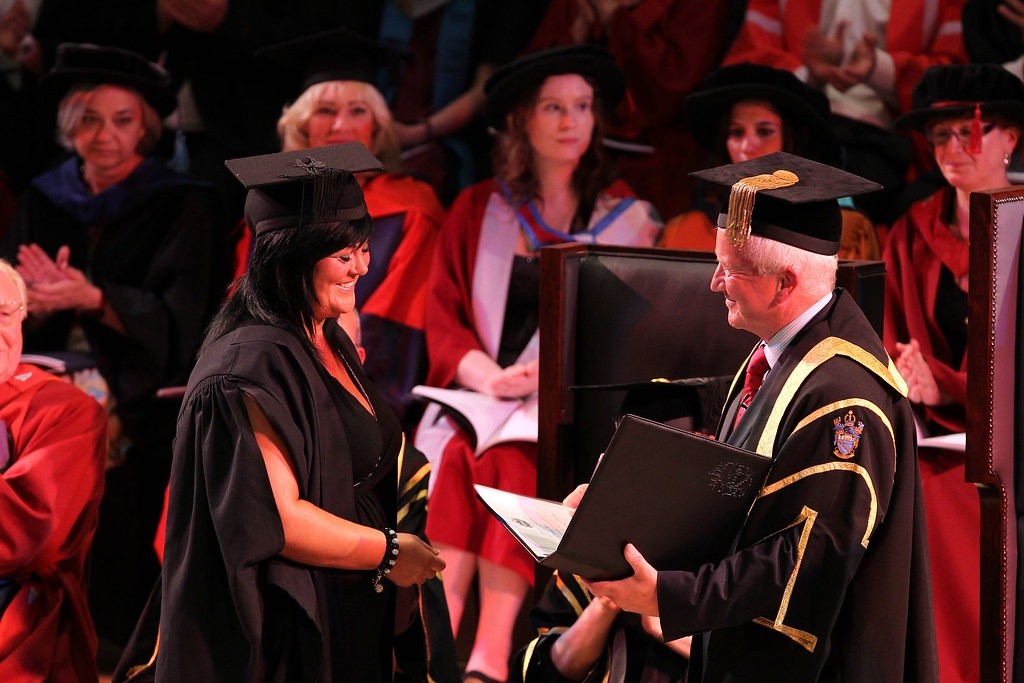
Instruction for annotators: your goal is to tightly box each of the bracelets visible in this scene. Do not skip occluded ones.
[369,527,398,596]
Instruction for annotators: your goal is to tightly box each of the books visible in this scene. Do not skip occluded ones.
[411,385,537,457]
[17,353,93,372]
[914,409,967,456]
[472,413,772,581]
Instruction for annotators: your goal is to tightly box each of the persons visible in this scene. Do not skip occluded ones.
[25,68,216,338]
[429,68,660,683]
[661,68,887,261]
[153,141,462,683]
[1,0,1022,179]
[562,151,942,682]
[0,256,105,683]
[234,68,443,323]
[881,69,1022,683]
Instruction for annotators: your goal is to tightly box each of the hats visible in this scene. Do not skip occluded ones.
[676,62,832,150]
[893,63,1023,157]
[686,153,887,254]
[224,142,385,237]
[302,57,381,90]
[41,48,181,120]
[482,43,625,119]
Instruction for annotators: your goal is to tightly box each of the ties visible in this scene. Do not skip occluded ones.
[731,344,769,431]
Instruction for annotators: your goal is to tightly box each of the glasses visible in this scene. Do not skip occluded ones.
[0,301,25,328]
